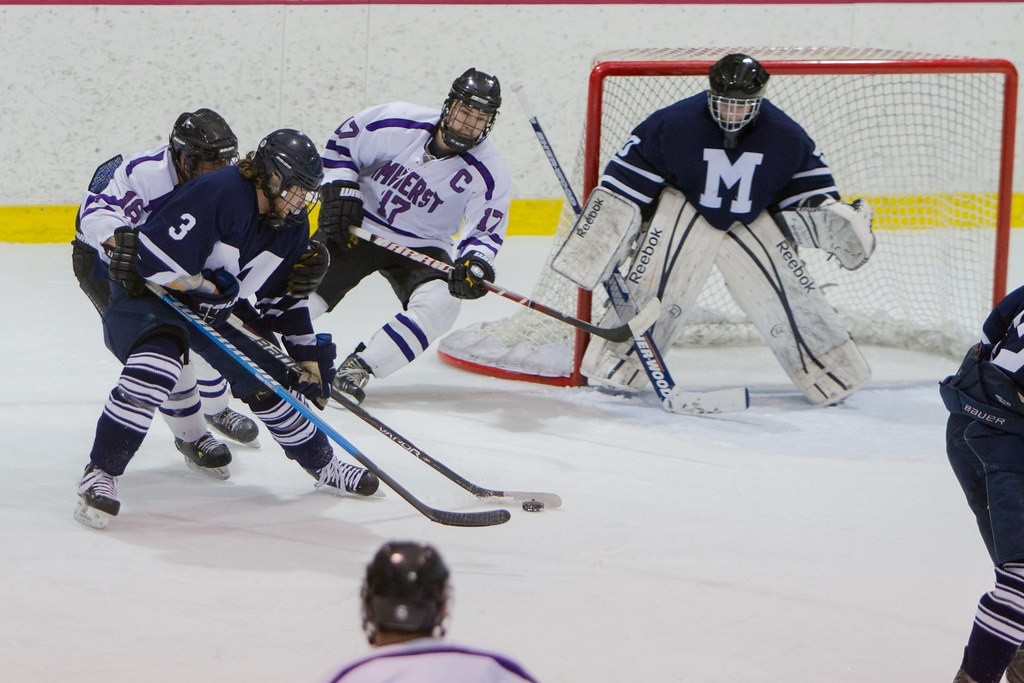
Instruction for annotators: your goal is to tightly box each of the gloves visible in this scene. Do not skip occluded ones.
[288,251,327,298]
[448,257,494,300]
[185,268,241,331]
[108,228,136,283]
[289,358,332,410]
[326,201,365,243]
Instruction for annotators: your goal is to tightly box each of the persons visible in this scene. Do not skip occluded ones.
[550,53,877,409]
[77,128,378,515]
[309,67,512,404]
[939,285,1024,683]
[73,111,259,467]
[318,541,538,683]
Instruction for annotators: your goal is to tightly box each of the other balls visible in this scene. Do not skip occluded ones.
[522,500,544,512]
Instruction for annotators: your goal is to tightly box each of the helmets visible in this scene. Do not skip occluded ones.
[255,129,326,229]
[439,67,501,153]
[708,53,769,132]
[171,109,239,185]
[360,542,450,646]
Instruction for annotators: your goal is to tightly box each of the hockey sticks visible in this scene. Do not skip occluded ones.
[343,226,663,345]
[144,274,564,528]
[502,79,751,417]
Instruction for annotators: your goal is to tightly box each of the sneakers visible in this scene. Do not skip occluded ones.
[204,405,261,450]
[72,459,120,529]
[330,343,373,404]
[176,430,232,480]
[309,452,387,498]
